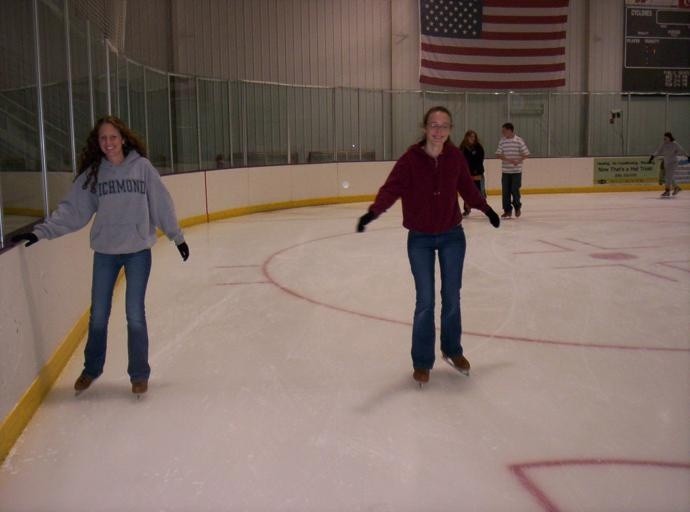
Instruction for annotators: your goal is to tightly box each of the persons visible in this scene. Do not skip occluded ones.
[648,133,690,196]
[495,122,530,216]
[459,130,486,215]
[356,105,500,380]
[12,115,189,392]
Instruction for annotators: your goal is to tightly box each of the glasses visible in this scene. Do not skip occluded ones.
[427,121,453,130]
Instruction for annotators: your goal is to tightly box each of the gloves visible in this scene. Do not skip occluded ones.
[10,232,39,247]
[177,242,190,262]
[356,211,375,232]
[648,155,654,164]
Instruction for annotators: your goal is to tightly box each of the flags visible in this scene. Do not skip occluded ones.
[415,0,569,91]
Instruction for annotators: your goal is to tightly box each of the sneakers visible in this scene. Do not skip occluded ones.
[661,191,670,196]
[501,212,512,217]
[515,207,521,217]
[441,348,471,370]
[672,185,681,195]
[486,206,501,228]
[73,370,94,391]
[130,376,149,396]
[462,210,471,216]
[413,367,431,383]
